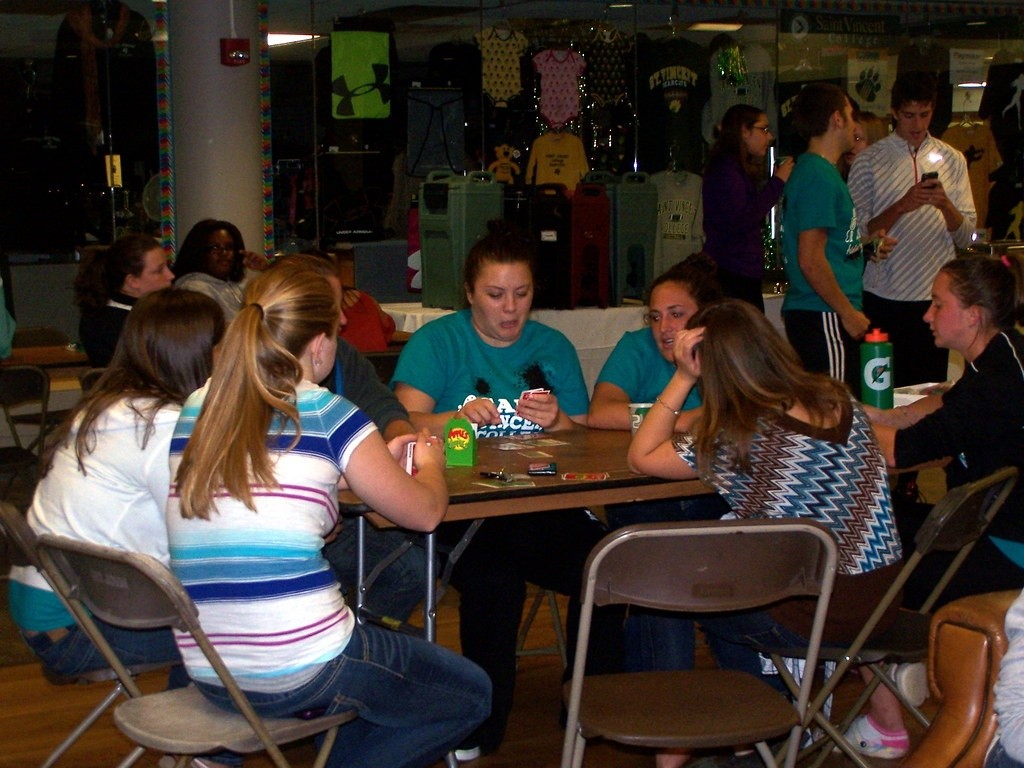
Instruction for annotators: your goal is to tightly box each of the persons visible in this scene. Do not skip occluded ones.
[780,82,898,403]
[389,234,626,760]
[78,233,174,368]
[847,70,977,388]
[338,285,396,352]
[627,296,902,768]
[813,254,1024,757]
[588,252,725,768]
[170,263,491,768]
[276,253,416,444]
[9,287,242,768]
[701,104,794,314]
[844,111,885,164]
[982,587,1024,768]
[171,219,269,320]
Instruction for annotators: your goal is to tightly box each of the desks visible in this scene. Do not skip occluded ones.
[328,413,719,768]
[0,345,87,457]
[374,291,786,402]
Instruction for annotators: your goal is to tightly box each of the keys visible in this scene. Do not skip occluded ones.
[481,465,514,482]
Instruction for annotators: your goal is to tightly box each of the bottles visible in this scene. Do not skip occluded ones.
[860,329,894,410]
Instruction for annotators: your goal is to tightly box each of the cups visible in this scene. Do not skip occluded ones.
[775,156,789,168]
[629,403,654,439]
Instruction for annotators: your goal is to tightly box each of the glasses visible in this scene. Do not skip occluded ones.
[746,124,770,135]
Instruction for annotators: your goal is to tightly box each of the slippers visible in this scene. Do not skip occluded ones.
[812,715,908,759]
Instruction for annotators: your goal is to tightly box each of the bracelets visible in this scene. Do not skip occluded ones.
[656,397,680,415]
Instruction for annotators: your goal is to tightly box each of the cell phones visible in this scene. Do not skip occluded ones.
[921,172,939,189]
[294,708,327,721]
[527,462,557,475]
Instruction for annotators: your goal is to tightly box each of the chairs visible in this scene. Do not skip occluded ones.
[0,243,1024,767]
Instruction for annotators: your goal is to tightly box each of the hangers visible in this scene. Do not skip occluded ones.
[961,96,977,129]
[490,7,689,204]
[18,126,69,154]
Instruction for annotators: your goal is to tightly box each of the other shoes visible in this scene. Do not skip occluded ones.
[190,757,232,768]
[728,749,767,768]
[889,661,930,708]
[453,734,480,761]
